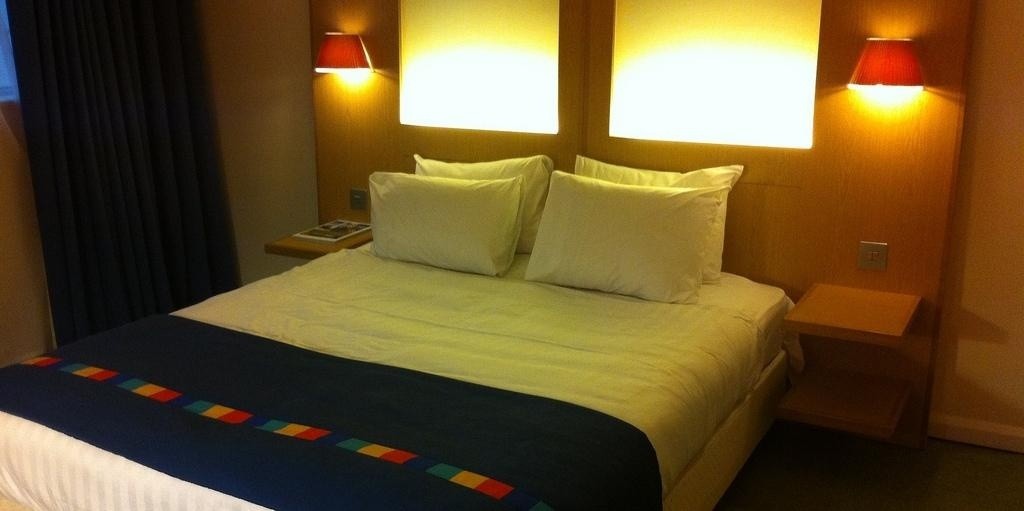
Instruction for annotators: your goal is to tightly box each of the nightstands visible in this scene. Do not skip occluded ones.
[265,225,367,257]
[780,283,923,445]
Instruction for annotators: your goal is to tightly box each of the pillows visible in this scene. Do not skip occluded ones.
[572,153,746,287]
[367,169,526,279]
[523,170,734,307]
[409,153,557,257]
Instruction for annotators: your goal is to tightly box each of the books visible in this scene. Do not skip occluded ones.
[294,219,369,243]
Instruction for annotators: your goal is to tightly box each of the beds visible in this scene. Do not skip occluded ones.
[0,221,790,511]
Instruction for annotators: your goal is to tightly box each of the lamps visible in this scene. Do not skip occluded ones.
[850,37,928,92]
[609,2,821,153]
[398,1,564,137]
[311,29,378,76]
